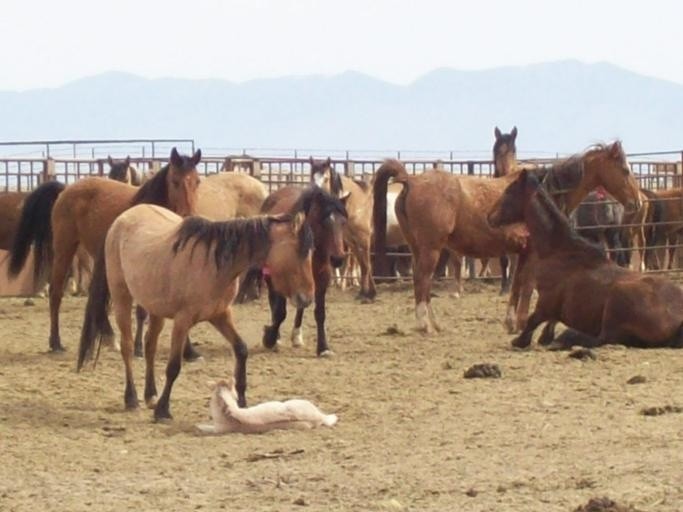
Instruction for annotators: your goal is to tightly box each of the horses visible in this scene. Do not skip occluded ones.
[0,191,86,297]
[205,376,338,433]
[485,166,683,351]
[0,146,202,357]
[195,170,270,301]
[372,140,643,337]
[491,127,521,296]
[576,188,683,273]
[105,154,158,187]
[310,154,409,304]
[327,182,494,291]
[231,183,352,357]
[75,201,320,423]
